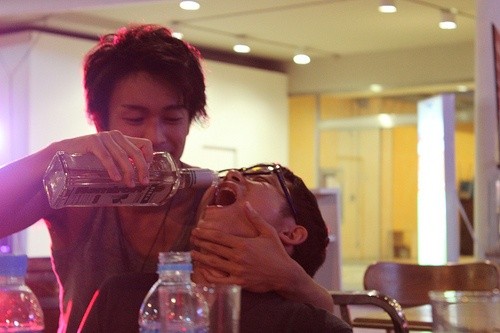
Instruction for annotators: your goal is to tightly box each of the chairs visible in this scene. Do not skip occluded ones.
[365,260,500,306]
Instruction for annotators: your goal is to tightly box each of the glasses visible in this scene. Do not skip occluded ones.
[216,164,301,225]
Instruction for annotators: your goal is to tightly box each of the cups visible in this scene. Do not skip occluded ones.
[155,283,241,333]
[432,287,500,333]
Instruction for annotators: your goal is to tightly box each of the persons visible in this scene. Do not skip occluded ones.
[0,24,334,333]
[77,163,354,333]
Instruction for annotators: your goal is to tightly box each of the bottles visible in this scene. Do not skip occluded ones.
[42,150,219,209]
[136,249,211,333]
[0,254,45,333]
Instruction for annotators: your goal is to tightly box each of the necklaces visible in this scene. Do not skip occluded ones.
[113,197,173,270]
